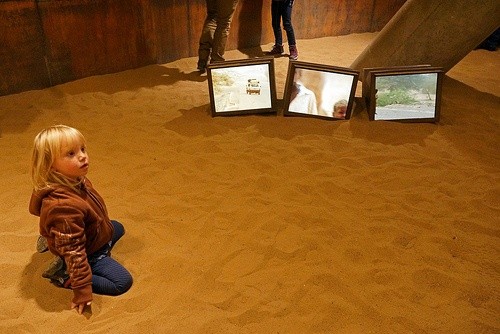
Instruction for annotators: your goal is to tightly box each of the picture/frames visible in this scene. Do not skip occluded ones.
[206,56,279,117]
[362,63,446,123]
[282,59,361,121]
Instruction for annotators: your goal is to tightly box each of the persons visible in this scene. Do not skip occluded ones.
[332,100,348,120]
[266,0,299,60]
[288,68,318,115]
[197,0,239,74]
[29,125,133,315]
